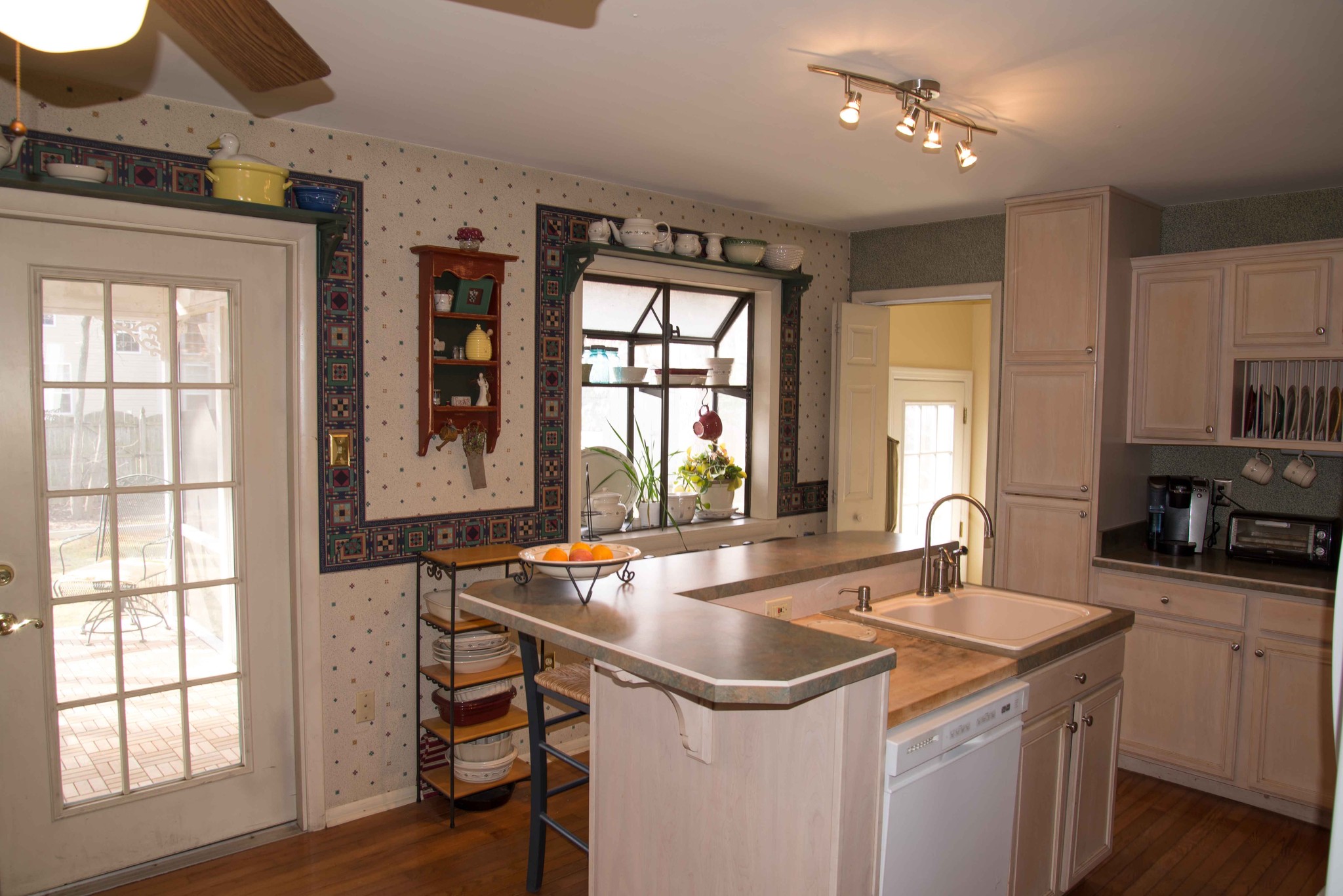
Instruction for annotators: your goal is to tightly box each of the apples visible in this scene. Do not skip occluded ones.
[569,548,595,561]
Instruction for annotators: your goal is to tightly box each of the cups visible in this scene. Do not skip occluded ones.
[693,405,723,441]
[582,363,592,383]
[1282,453,1318,488]
[631,517,642,529]
[434,294,453,313]
[1241,451,1274,485]
[707,358,734,385]
[667,491,699,524]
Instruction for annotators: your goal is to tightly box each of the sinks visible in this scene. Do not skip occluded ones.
[850,582,1113,651]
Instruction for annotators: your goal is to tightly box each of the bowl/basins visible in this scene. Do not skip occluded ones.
[518,542,641,580]
[46,163,108,184]
[431,631,517,673]
[613,367,707,384]
[292,185,345,213]
[445,731,518,783]
[721,238,805,272]
[424,588,485,623]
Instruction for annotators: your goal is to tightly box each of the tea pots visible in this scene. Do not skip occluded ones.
[608,213,671,252]
[0,127,28,168]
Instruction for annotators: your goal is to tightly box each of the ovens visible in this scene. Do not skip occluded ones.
[1225,509,1342,572]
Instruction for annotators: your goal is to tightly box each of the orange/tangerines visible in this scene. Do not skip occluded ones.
[569,542,592,553]
[591,544,613,560]
[543,547,568,561]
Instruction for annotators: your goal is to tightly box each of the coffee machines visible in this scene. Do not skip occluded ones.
[1146,475,1210,557]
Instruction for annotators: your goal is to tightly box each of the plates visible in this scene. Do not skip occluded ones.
[581,446,639,514]
[1244,383,1343,442]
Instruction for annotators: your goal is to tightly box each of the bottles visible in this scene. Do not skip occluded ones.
[582,345,622,384]
[583,487,626,533]
[465,323,492,361]
[433,389,441,406]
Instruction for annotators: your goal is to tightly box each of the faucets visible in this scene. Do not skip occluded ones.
[915,492,994,599]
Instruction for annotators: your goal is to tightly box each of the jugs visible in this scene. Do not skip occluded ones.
[588,218,611,245]
[654,231,674,253]
[674,233,702,258]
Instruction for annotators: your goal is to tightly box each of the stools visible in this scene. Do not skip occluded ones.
[518,631,590,895]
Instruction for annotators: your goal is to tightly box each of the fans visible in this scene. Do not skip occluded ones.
[152,0,330,94]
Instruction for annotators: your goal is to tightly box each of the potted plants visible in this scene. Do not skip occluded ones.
[583,414,690,553]
[672,441,748,511]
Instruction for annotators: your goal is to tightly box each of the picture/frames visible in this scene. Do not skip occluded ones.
[454,278,494,314]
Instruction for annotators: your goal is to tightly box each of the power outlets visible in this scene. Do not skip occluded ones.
[355,690,375,724]
[1211,477,1232,507]
[764,596,793,621]
[544,651,555,670]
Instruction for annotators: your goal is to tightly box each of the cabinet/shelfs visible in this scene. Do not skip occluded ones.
[415,543,532,829]
[991,189,1343,830]
[1006,630,1126,896]
[409,244,520,457]
[559,272,755,539]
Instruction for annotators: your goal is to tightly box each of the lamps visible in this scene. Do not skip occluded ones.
[810,64,997,168]
[0,0,150,136]
